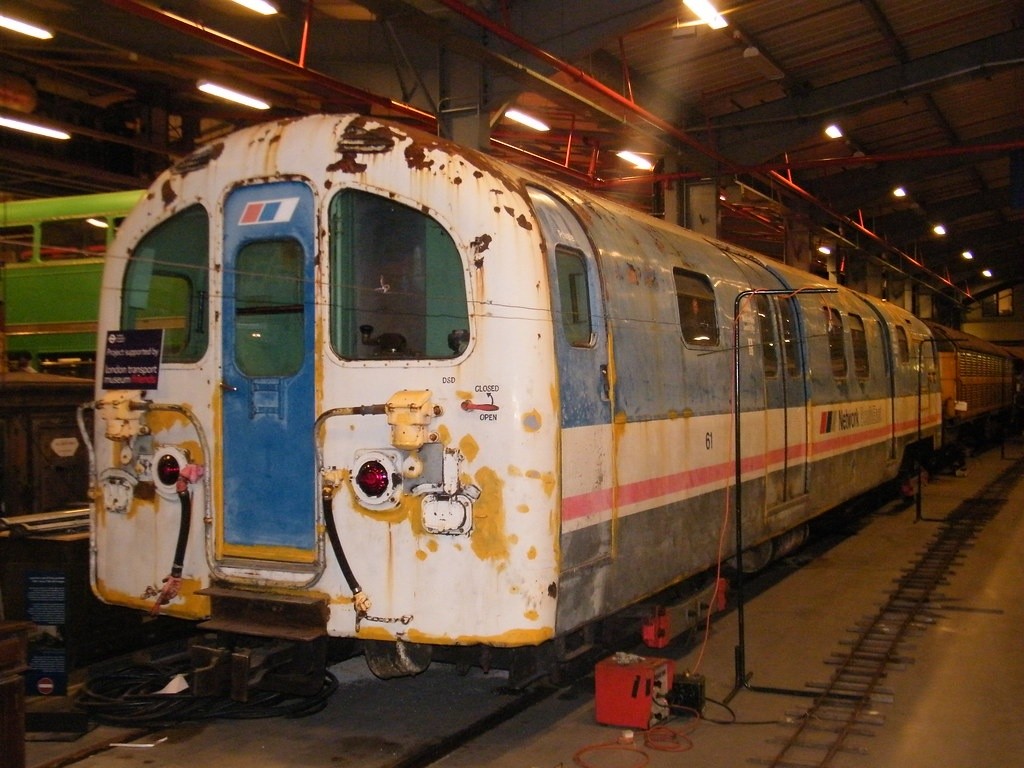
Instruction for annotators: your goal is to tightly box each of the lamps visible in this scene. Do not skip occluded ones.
[744,46,784,81]
[616,150,654,169]
[683,0,729,30]
[232,0,280,15]
[0,11,56,41]
[0,112,72,141]
[195,75,274,110]
[505,104,551,132]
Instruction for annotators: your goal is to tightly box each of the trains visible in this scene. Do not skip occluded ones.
[74,110,1024,694]
